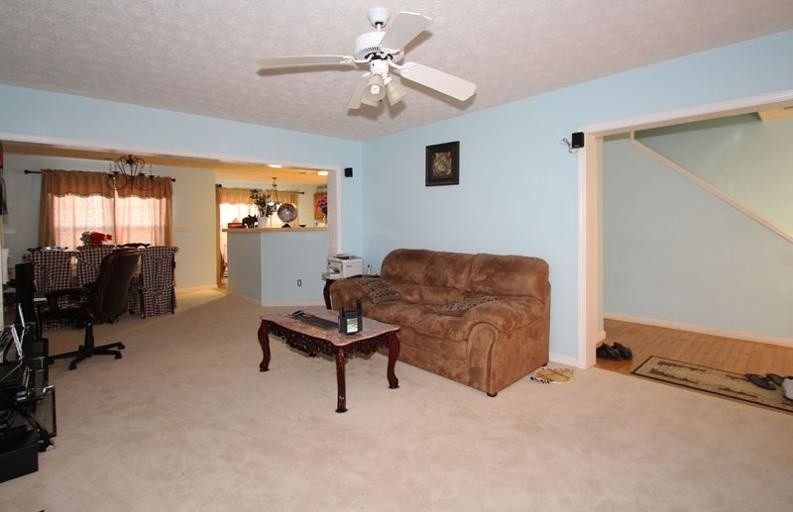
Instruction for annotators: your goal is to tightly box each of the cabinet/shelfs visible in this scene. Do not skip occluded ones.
[223,228,328,306]
[0,321,51,484]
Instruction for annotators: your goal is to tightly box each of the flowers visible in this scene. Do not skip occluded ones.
[80,230,113,248]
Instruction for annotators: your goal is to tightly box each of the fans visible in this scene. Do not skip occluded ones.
[257,8,478,110]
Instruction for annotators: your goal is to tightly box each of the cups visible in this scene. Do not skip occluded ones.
[313,219,318,228]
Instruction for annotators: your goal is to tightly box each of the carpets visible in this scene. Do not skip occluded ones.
[630,354,793,412]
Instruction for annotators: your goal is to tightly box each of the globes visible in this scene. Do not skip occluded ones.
[278,203,297,228]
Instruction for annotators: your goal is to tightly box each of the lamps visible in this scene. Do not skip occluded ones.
[109,155,157,191]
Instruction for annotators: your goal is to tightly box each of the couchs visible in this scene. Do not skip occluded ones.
[330,249,551,397]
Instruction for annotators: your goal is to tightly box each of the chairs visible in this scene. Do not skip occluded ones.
[130,247,179,319]
[78,249,114,288]
[38,242,144,370]
[29,250,73,329]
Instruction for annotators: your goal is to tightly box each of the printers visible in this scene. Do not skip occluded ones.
[321,254,363,281]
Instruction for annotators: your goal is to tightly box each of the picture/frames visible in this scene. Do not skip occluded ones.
[425,142,459,185]
